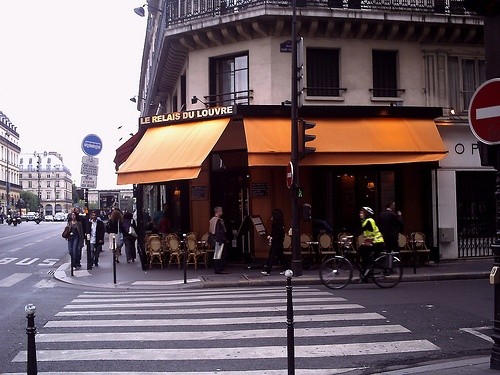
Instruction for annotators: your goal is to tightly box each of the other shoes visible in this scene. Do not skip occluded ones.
[261,271,285,276]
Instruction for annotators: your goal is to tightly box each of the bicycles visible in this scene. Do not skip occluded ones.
[319,237,404,290]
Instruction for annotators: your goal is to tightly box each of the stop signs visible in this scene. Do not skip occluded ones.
[468,78,500,144]
[286,161,294,188]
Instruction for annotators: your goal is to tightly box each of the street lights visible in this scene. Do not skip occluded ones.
[6,132,10,209]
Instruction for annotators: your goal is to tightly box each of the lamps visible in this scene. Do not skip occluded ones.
[367,182,374,190]
[190,95,210,109]
[134,4,163,21]
[130,94,146,106]
[173,188,181,197]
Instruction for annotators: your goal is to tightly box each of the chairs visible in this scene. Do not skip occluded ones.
[282,233,431,265]
[144,231,216,269]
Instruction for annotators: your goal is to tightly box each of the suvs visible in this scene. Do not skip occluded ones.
[54,213,64,221]
[26,212,36,222]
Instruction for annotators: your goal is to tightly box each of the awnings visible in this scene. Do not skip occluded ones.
[113,129,146,172]
[243,118,450,167]
[116,118,231,185]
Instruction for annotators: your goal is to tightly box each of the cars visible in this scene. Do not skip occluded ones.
[45,215,53,221]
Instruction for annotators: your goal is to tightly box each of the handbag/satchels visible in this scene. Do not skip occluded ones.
[362,238,373,247]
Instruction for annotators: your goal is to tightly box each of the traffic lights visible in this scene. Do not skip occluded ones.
[296,118,317,160]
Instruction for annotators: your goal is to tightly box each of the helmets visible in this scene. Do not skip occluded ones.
[363,206,374,216]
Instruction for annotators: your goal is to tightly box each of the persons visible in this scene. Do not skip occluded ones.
[208,207,231,273]
[1,210,42,224]
[71,207,85,266]
[260,208,287,276]
[122,212,138,263]
[343,206,384,283]
[66,212,80,269]
[85,212,105,267]
[106,210,125,263]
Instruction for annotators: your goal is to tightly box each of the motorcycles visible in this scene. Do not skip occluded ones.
[5,213,43,226]
